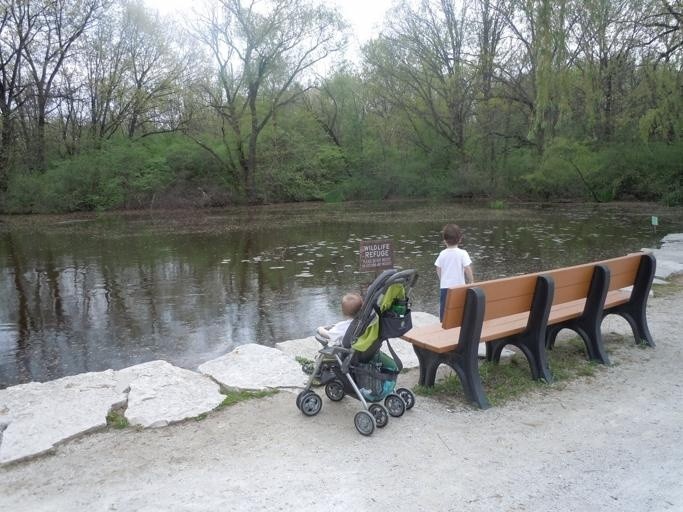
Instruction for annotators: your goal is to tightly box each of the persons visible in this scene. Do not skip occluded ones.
[434,223,473,324]
[326,292,363,349]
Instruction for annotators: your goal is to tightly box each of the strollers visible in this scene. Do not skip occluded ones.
[296,265,418,437]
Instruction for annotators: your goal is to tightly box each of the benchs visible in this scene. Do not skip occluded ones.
[399,252,657,410]
